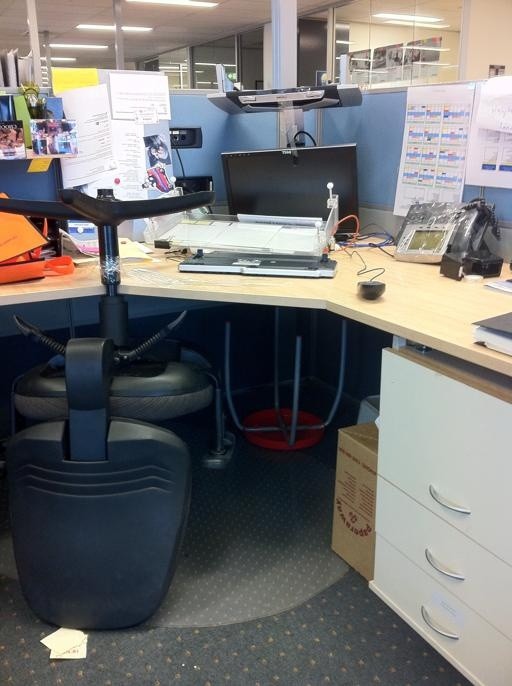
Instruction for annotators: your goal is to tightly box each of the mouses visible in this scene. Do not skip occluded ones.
[359,281,386,300]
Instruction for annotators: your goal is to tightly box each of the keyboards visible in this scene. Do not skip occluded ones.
[178,251,337,279]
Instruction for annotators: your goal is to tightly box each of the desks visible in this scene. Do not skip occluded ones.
[1,235,512,686]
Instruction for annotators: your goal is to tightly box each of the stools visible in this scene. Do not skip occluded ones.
[225,319,347,450]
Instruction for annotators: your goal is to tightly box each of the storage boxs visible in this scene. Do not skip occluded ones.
[330,423,378,582]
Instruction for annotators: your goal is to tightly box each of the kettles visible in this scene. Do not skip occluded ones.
[446,195,503,279]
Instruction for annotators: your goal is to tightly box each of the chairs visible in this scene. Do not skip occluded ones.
[1,188,235,632]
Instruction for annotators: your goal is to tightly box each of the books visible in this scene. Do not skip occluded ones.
[58,227,154,268]
[472,311,512,358]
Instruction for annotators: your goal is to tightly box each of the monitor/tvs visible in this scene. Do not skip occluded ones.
[0,158,68,243]
[221,143,361,242]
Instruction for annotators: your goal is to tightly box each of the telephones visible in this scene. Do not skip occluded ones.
[394,197,501,264]
